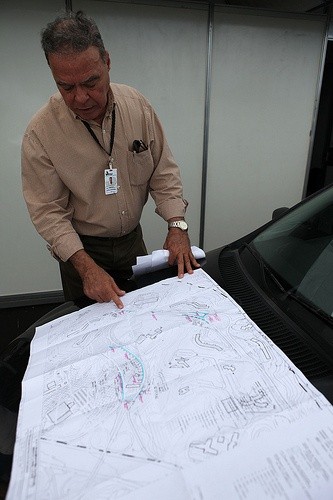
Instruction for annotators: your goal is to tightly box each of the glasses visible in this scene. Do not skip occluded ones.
[131,139,150,153]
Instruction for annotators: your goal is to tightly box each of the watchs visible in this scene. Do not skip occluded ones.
[167,220,188,231]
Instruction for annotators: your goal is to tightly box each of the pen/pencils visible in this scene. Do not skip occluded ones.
[132,151,136,163]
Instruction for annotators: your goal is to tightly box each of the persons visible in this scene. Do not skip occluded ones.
[20,13,201,309]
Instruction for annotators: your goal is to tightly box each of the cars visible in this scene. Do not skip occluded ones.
[0,184,333,500]
